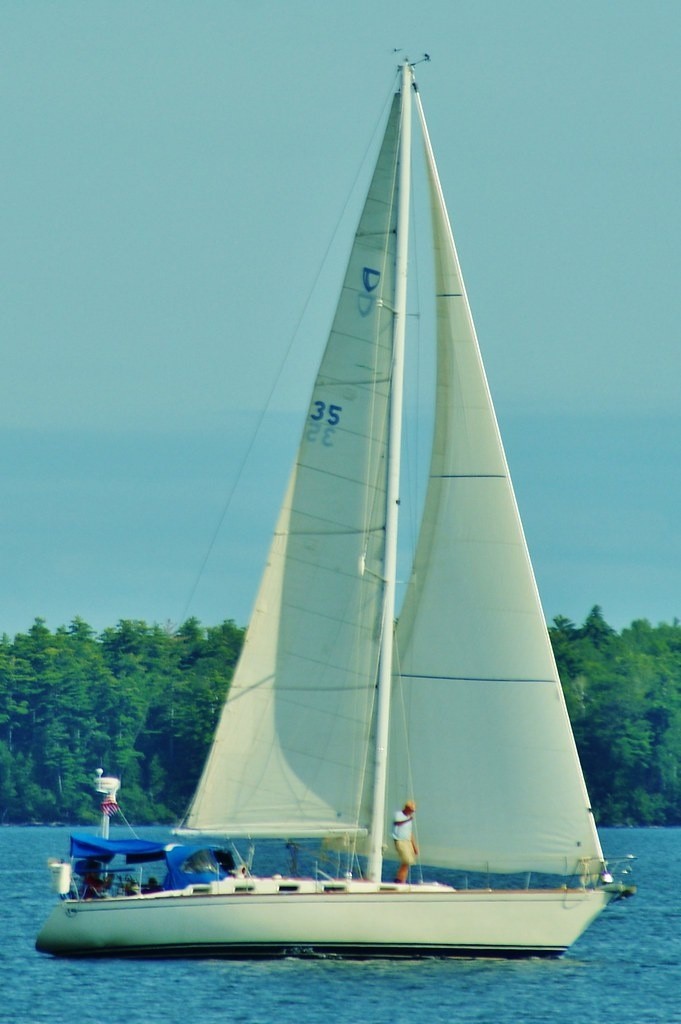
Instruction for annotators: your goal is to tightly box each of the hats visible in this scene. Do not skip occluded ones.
[405,801,415,811]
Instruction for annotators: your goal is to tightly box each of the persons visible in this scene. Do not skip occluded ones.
[392,799,421,884]
[100,873,122,902]
[80,863,104,900]
[234,864,248,882]
[144,875,164,895]
[85,879,108,903]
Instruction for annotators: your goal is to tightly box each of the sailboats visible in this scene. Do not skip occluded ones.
[30,50,640,960]
